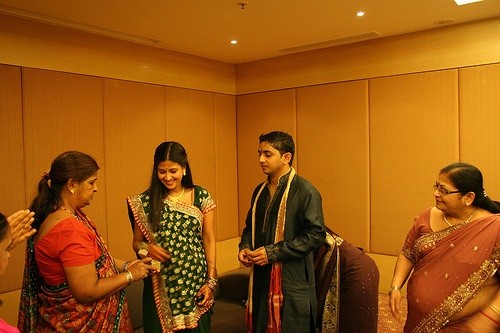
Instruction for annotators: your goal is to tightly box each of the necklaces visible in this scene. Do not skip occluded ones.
[167,187,185,199]
[443,207,479,230]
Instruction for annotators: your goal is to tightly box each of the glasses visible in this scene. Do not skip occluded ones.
[433,184,461,195]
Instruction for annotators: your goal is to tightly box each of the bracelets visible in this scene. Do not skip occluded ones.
[123,260,133,272]
[206,275,219,293]
[124,270,132,286]
[390,287,400,291]
[489,303,500,314]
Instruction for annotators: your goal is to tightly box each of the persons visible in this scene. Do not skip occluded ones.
[126,141,217,333]
[17,151,160,332]
[0,209,38,332]
[239,132,326,333]
[389,162,500,333]
